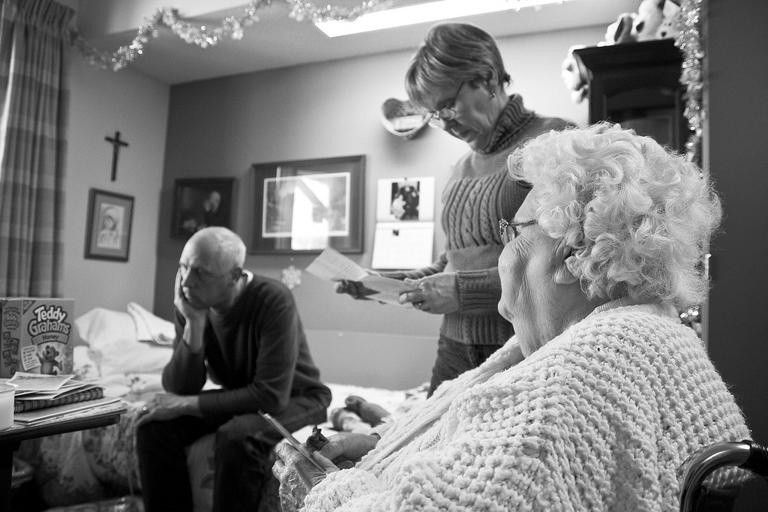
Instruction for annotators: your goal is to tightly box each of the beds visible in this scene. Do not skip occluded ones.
[11,373,432,511]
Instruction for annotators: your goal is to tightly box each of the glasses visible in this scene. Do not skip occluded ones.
[423,80,466,122]
[494,216,547,241]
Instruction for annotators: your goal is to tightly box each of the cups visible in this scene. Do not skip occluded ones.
[0,381,17,430]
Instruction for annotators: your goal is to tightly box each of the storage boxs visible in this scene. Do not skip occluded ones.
[0,296,77,378]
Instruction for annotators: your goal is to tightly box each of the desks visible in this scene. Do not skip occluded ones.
[0,388,128,510]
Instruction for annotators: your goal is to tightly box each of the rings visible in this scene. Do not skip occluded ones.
[417,301,423,310]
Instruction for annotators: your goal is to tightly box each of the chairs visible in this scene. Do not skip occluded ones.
[679,438,768,512]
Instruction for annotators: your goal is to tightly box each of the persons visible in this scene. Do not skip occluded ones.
[96,206,122,249]
[392,180,421,216]
[134,225,332,511]
[266,120,758,512]
[335,21,579,399]
[200,190,225,226]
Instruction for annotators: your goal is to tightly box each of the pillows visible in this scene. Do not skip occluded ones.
[71,307,177,396]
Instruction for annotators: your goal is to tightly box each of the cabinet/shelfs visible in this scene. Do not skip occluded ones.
[571,36,698,156]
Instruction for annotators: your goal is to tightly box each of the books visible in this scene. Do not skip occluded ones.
[3,369,129,427]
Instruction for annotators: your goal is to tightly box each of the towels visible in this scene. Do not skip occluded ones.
[122,301,179,349]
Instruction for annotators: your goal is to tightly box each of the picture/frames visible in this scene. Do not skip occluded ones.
[81,186,136,264]
[245,152,368,256]
[169,176,240,244]
